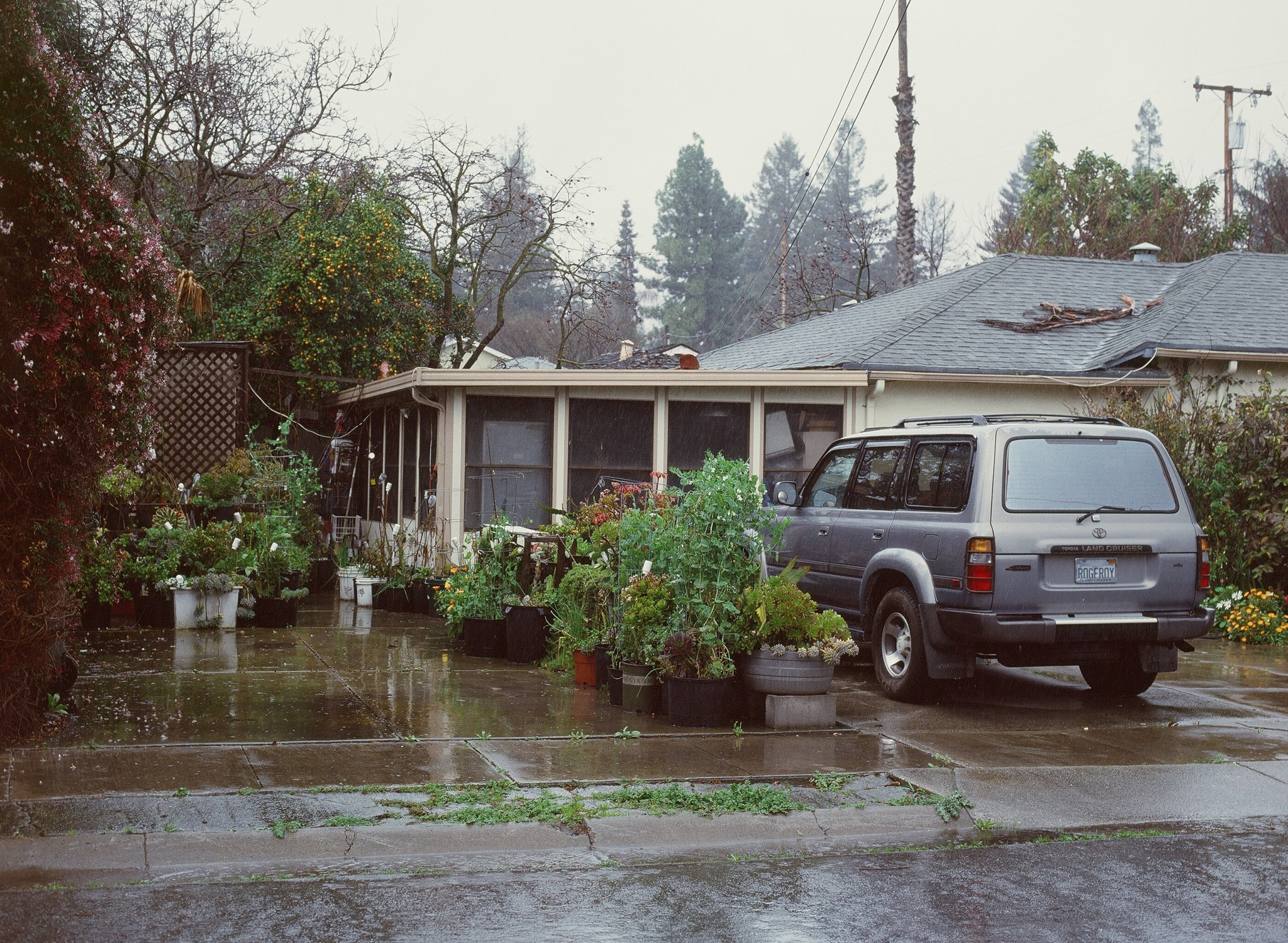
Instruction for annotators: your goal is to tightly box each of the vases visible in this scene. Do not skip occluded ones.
[464,618,505,657]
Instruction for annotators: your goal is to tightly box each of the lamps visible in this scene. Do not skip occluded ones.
[400,408,411,418]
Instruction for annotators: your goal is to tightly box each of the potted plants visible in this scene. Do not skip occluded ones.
[504,449,859,725]
[78,412,322,629]
[335,535,446,612]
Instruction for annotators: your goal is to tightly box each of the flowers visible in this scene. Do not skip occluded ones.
[621,572,671,604]
[432,566,483,636]
[575,470,676,526]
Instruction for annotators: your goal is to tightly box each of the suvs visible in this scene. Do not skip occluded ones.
[743,414,1216,702]
